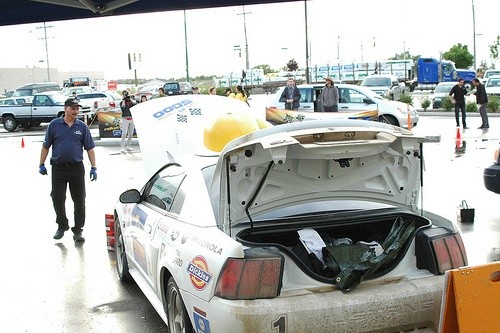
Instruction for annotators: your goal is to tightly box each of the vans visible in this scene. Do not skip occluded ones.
[162,81,195,98]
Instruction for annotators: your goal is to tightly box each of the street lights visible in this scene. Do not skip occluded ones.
[237,11,252,74]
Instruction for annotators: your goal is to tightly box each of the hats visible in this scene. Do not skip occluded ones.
[223,88,231,94]
[64,99,83,107]
[325,77,333,81]
[72,92,76,94]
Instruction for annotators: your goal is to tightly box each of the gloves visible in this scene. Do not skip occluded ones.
[90,166,97,182]
[39,164,47,175]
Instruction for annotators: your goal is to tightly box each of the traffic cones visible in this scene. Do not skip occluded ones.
[21,138,25,148]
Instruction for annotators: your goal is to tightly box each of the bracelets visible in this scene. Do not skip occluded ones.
[91,166,96,168]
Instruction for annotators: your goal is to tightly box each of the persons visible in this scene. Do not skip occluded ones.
[279,78,302,111]
[399,80,414,94]
[119,90,137,154]
[38,97,98,244]
[131,88,169,103]
[192,85,248,102]
[321,77,339,112]
[472,78,490,129]
[71,91,80,102]
[448,78,470,129]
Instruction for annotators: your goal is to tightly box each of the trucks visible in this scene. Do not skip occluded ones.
[312,59,479,92]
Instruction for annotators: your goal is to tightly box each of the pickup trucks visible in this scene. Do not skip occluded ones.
[0,77,122,132]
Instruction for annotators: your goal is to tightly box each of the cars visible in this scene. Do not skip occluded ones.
[431,81,464,110]
[359,76,403,102]
[218,78,262,85]
[481,69,500,98]
[105,84,468,333]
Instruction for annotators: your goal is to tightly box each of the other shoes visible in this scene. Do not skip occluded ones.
[54,225,70,239]
[73,234,85,243]
[120,147,124,152]
[127,148,133,151]
